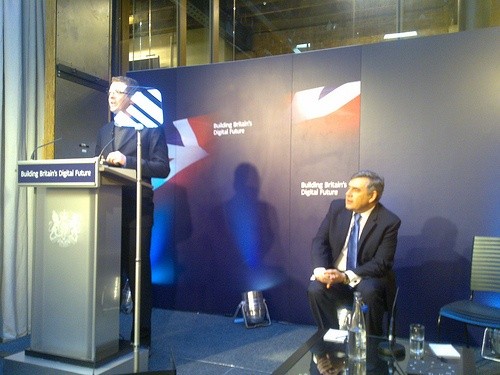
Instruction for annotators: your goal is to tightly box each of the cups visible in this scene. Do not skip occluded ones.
[409,324,424,358]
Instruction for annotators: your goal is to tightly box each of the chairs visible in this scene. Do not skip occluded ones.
[437,235,500,349]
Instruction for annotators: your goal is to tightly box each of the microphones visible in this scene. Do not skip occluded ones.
[31,138,63,160]
[99,136,115,158]
[378,287,405,357]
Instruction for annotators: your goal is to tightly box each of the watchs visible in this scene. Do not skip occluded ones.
[343,272,350,285]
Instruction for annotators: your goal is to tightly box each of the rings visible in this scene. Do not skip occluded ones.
[328,275,331,279]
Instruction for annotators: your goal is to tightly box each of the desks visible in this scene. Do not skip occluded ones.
[285,328,500,375]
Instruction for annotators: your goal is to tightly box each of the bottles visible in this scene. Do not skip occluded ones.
[348,292,366,358]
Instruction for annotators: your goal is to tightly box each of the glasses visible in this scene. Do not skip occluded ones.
[107,91,128,96]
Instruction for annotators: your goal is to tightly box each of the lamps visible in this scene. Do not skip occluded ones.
[232,289,271,329]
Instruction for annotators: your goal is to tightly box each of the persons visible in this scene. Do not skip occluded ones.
[93,75,170,359]
[306,171,402,333]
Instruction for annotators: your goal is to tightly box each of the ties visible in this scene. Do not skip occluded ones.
[346,213,361,290]
